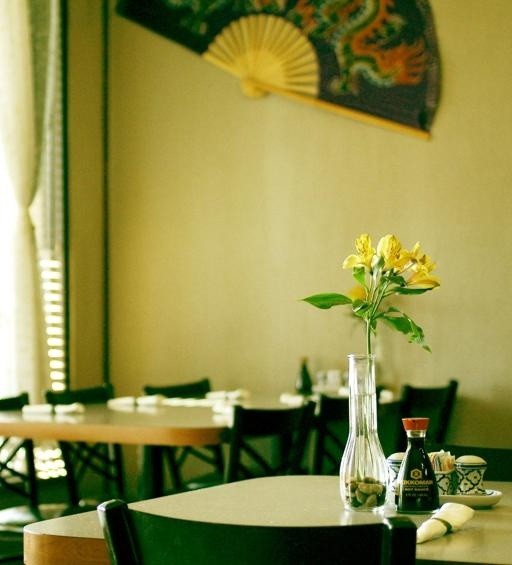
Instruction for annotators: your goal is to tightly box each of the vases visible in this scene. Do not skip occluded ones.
[339,355,392,511]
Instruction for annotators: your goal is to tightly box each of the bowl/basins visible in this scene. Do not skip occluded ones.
[433,465,457,495]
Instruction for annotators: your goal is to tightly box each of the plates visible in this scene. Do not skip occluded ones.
[437,489,503,509]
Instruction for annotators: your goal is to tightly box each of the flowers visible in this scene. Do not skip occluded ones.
[298,233,441,441]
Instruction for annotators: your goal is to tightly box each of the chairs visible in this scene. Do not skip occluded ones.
[144,380,223,495]
[0,393,46,561]
[98,494,419,563]
[47,383,130,516]
[394,381,458,457]
[219,398,317,483]
[310,386,383,474]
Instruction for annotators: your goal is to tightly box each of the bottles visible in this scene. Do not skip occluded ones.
[454,455,487,496]
[387,452,406,494]
[295,357,313,396]
[397,418,439,515]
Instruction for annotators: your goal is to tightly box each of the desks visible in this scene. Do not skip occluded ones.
[19,472,512,563]
[1,378,405,465]
